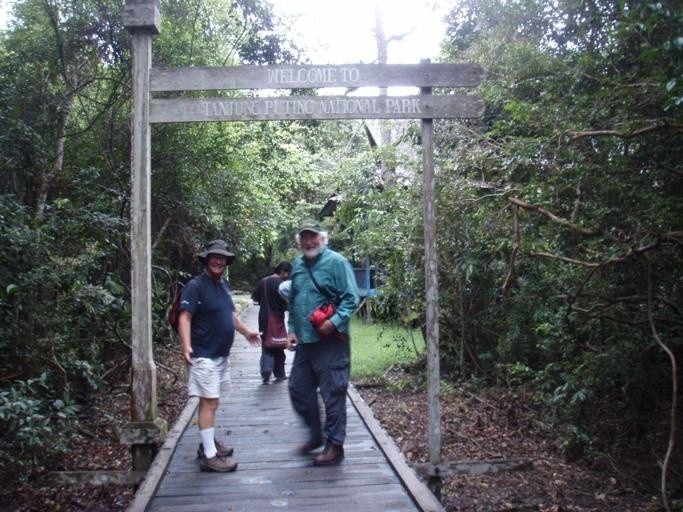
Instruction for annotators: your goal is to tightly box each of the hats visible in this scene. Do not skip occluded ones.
[197,239,235,267]
[297,219,321,236]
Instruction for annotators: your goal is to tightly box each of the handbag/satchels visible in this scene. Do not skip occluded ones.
[309,301,336,340]
[264,313,289,351]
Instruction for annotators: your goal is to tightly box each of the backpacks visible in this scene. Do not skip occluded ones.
[170,275,206,333]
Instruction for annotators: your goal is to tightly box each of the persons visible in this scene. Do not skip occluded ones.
[176,238,264,473]
[283,218,361,467]
[278,278,321,394]
[251,261,293,385]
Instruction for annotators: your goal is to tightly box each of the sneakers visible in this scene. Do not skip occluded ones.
[200,452,237,473]
[197,437,233,458]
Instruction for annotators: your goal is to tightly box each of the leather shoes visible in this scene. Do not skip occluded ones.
[313,440,344,467]
[298,437,323,454]
